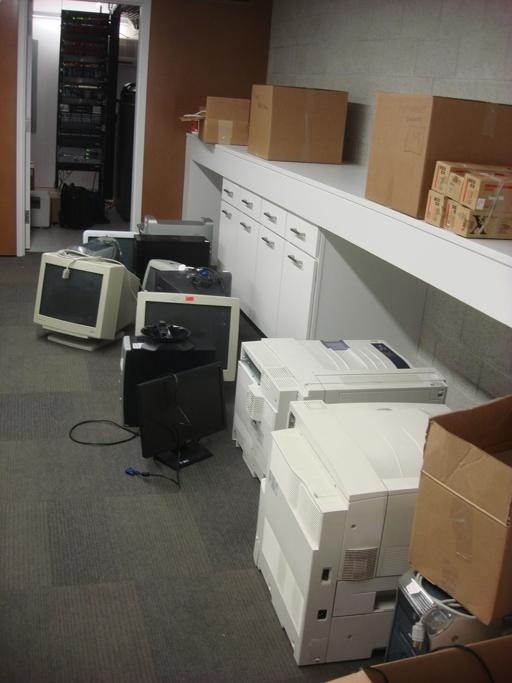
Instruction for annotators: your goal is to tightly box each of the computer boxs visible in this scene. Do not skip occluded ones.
[139,214,214,240]
[384,568,511,665]
[119,336,216,427]
[133,234,210,285]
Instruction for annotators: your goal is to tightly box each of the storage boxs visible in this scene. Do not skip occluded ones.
[326,634,512,682]
[363,92,512,238]
[200,84,347,165]
[406,393,512,627]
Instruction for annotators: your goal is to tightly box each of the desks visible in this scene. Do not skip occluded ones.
[181,131,512,412]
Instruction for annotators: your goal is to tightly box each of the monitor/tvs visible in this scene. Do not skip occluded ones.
[83,230,140,275]
[134,291,240,382]
[155,270,231,297]
[135,361,226,470]
[33,252,140,352]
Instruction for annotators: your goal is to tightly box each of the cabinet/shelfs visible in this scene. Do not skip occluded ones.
[213,176,428,357]
